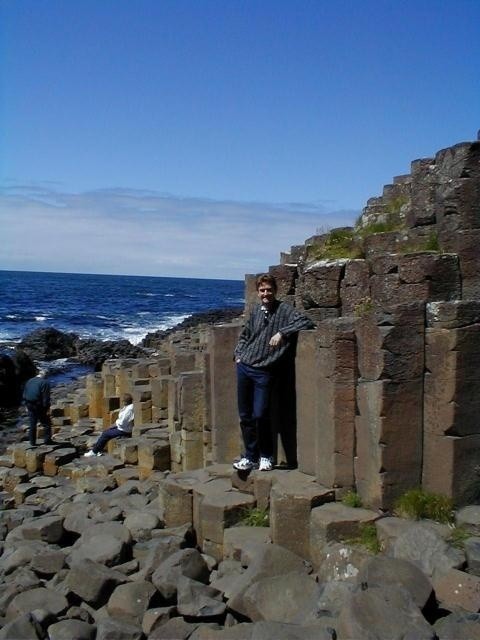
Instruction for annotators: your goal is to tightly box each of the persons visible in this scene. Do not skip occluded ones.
[22,369,58,446]
[232,273,313,472]
[84,394,133,458]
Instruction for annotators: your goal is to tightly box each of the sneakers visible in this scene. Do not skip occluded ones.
[31,441,36,446]
[84,448,103,457]
[232,456,257,471]
[45,440,58,445]
[259,458,274,472]
